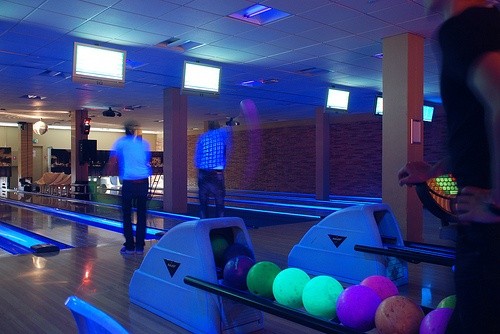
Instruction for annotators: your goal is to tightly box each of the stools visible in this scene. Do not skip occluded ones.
[34,172,71,208]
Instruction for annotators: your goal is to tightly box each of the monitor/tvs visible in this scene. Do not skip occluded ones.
[72,41,127,88]
[373,94,384,117]
[180,59,223,98]
[422,103,435,124]
[322,86,351,114]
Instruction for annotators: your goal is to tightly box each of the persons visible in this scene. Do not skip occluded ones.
[106,120,153,255]
[396,0,500,334]
[194,119,232,218]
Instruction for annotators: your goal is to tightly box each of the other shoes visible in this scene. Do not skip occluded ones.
[135,248,144,255]
[120,246,134,255]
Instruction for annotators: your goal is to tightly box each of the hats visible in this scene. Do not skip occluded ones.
[208,120,220,129]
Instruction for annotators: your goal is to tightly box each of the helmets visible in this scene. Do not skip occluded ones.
[123,120,139,130]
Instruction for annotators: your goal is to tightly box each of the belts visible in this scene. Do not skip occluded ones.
[212,171,223,174]
[132,181,145,184]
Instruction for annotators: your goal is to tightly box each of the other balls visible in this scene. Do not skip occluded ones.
[360,275,399,301]
[436,295,456,308]
[272,268,310,309]
[211,238,228,262]
[420,308,453,334]
[375,296,424,334]
[302,275,345,321]
[221,244,254,268]
[246,261,281,299]
[336,285,381,333]
[223,256,256,290]
